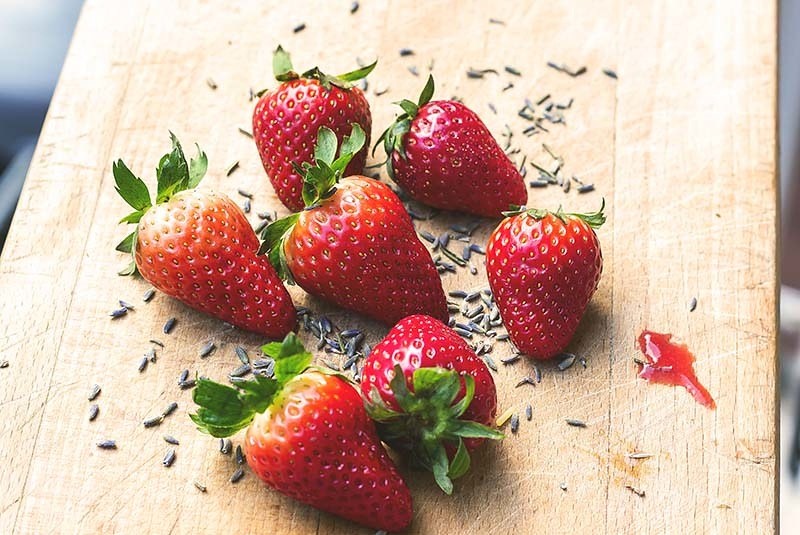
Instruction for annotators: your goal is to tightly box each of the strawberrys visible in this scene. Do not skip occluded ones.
[253,44,379,211]
[187,333,413,532]
[360,316,507,496]
[484,197,607,360]
[112,128,297,339]
[253,123,452,327]
[369,73,528,219]
